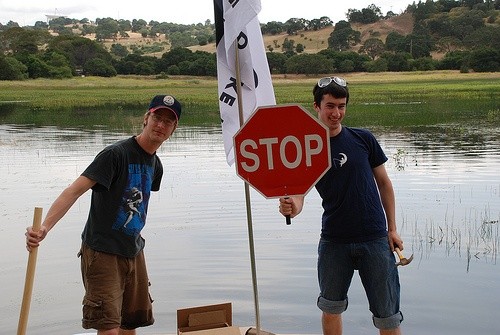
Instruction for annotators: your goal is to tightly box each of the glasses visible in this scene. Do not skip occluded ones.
[315,76,348,88]
[149,114,177,126]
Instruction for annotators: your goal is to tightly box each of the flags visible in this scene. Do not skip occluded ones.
[213,0,279,170]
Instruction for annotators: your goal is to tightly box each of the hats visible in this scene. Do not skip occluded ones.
[148,95,181,125]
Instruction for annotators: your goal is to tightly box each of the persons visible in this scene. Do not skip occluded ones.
[25,94,183,335]
[278,76,404,335]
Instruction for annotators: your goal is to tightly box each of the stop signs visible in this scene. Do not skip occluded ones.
[232,104,332,199]
